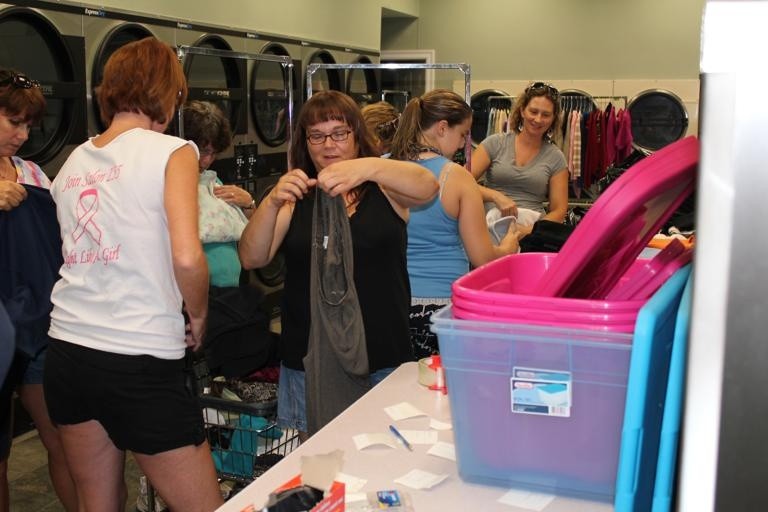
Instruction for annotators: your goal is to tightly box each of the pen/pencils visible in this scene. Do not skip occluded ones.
[390,424,412,450]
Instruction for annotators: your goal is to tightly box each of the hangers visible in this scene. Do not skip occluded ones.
[570,96,573,112]
[583,95,625,111]
[575,96,578,112]
[567,96,569,112]
[580,95,582,112]
[487,95,519,113]
[563,96,566,112]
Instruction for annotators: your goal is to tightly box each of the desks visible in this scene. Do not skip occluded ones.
[215,362,614,512]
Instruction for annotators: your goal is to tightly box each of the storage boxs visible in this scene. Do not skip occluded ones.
[437,152,685,483]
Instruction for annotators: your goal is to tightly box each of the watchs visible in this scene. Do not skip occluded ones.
[242,199,255,210]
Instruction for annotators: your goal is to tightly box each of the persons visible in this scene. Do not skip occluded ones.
[382,88,522,361]
[359,102,401,156]
[0,65,77,511]
[240,90,441,443]
[47,39,224,512]
[173,99,258,290]
[463,82,569,241]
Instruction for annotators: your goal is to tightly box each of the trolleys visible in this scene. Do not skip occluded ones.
[185,348,300,484]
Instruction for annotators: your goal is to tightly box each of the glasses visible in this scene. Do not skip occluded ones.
[0,73,42,91]
[527,82,560,100]
[303,129,354,144]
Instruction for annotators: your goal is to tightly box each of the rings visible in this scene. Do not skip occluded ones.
[231,192,234,197]
